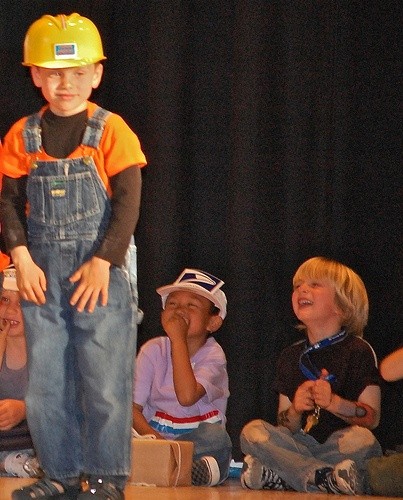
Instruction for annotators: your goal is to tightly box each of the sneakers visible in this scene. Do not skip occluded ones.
[318,459,364,496]
[192,456,220,487]
[241,454,287,490]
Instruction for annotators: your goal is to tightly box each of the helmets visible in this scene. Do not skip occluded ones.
[21,13,107,68]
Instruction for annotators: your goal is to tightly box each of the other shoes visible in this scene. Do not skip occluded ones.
[23,456,44,478]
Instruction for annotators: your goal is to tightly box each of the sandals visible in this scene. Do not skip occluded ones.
[12,477,81,500]
[77,473,125,500]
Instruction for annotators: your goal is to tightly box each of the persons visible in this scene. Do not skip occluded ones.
[0,263,43,477]
[132,268,231,486]
[0,13,147,500]
[240,257,392,497]
[368,348,403,497]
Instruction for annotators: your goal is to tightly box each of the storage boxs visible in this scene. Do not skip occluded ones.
[127,438,193,486]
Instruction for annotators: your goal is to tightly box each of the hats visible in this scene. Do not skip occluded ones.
[2,268,19,291]
[156,269,227,321]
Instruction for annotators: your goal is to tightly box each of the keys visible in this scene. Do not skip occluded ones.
[303,415,318,433]
